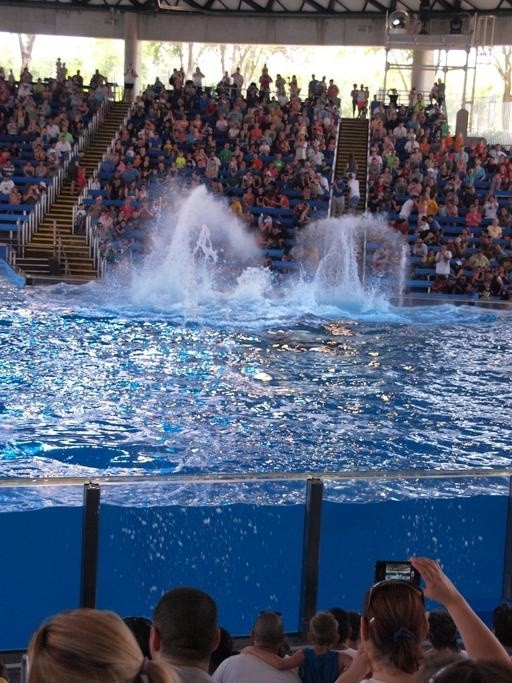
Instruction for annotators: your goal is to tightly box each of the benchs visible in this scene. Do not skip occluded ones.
[365,188,512,300]
[0,129,66,236]
[80,182,327,277]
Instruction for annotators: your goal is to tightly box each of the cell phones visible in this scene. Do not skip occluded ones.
[374,557,424,587]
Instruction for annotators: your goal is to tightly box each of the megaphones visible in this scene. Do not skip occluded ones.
[388,10,410,35]
